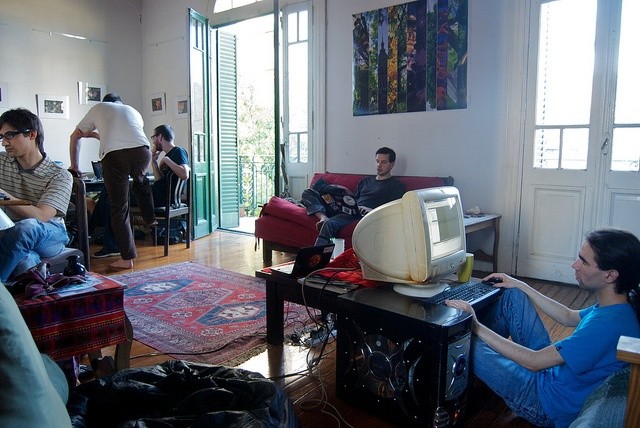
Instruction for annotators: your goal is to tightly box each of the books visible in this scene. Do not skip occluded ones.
[297,274,359,293]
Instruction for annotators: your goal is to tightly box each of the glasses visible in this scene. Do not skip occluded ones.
[0,128,30,140]
[151,134,157,141]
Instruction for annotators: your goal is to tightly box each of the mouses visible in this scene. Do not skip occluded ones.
[482,277,503,285]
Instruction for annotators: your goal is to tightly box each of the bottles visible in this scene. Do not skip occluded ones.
[63,254,85,278]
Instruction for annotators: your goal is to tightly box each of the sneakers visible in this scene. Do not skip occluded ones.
[92,248,120,258]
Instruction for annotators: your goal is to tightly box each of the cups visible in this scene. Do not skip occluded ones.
[457,253,475,283]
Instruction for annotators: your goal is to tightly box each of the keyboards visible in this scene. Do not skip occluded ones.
[428,281,500,306]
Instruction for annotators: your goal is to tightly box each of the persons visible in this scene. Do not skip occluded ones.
[67,93,152,268]
[302,147,405,247]
[1,280,73,428]
[1,108,73,284]
[93,125,189,258]
[444,228,639,426]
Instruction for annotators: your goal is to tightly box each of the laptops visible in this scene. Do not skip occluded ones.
[270,243,335,278]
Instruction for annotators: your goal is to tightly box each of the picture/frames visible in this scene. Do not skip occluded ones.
[174,95,189,120]
[78,81,106,105]
[150,93,166,115]
[36,93,71,119]
[0,82,9,107]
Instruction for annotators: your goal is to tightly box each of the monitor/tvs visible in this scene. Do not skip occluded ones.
[352,187,468,299]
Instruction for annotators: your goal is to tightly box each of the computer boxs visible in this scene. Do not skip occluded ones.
[338,288,474,428]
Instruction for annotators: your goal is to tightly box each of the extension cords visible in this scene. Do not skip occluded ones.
[305,329,337,346]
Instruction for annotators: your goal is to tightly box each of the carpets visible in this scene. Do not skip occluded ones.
[107,260,324,367]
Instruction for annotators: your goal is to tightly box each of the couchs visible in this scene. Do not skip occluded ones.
[254,172,454,266]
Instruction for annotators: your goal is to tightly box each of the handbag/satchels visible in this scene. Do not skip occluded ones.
[67,360,294,427]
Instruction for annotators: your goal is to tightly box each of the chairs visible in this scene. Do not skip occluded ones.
[131,171,193,256]
[67,177,92,271]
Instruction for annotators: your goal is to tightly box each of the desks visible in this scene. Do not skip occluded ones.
[465,211,502,272]
[15,268,134,370]
[255,260,504,427]
